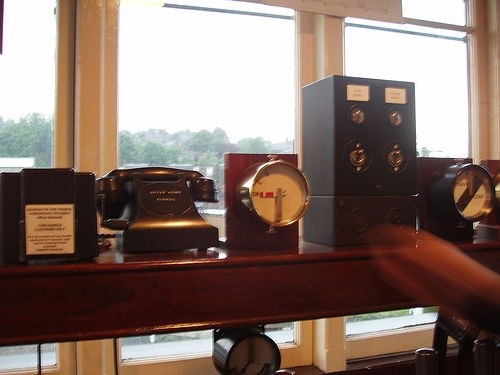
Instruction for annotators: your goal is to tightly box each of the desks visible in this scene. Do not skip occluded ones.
[0,226,500,375]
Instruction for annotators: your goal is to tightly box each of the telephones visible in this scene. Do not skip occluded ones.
[95,167,223,251]
